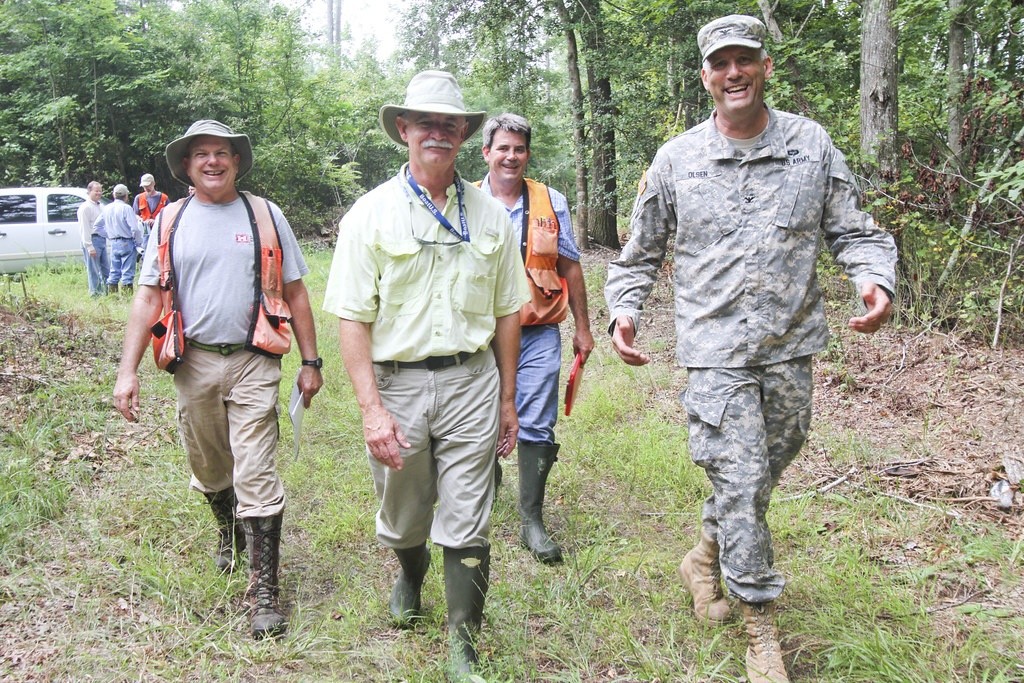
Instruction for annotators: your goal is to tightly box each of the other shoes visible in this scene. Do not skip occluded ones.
[121,285,133,295]
[108,285,118,294]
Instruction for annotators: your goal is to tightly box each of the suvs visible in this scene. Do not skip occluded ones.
[0,188,116,274]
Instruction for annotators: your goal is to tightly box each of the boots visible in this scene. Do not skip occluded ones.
[443,544,491,683]
[204,485,248,574]
[740,600,789,683]
[681,527,734,625]
[517,443,561,563]
[243,516,285,637]
[389,540,431,625]
[491,454,502,512]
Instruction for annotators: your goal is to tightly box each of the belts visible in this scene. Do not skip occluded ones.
[372,352,470,371]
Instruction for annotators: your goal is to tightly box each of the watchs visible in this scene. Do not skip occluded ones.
[302,358,323,369]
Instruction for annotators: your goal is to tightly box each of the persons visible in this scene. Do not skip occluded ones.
[77,174,198,298]
[113,119,324,638]
[469,114,594,564]
[604,15,898,683]
[322,71,532,683]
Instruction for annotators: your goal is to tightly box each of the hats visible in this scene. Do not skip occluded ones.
[113,184,132,196]
[139,173,154,187]
[697,14,766,63]
[378,70,488,147]
[165,120,254,186]
